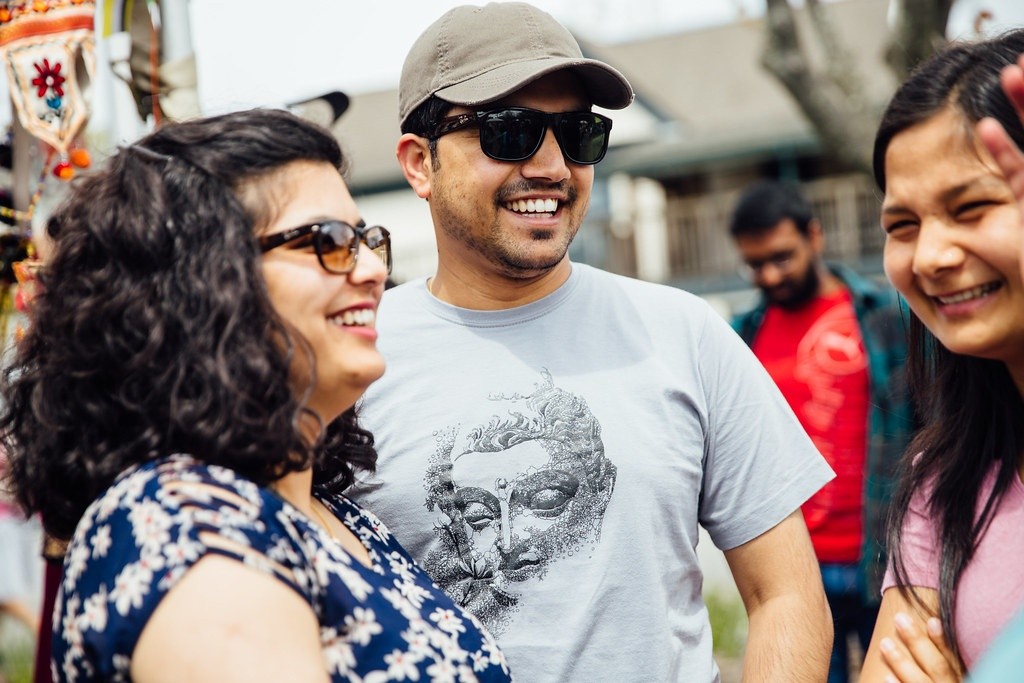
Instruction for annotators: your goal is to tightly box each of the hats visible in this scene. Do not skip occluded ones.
[393,2,634,132]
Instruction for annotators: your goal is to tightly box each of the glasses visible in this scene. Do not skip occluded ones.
[247,220,393,276]
[419,106,614,165]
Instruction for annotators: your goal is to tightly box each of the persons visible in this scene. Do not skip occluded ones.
[0,109,512,683]
[856,27,1024,683]
[334,0,839,683]
[724,178,950,683]
